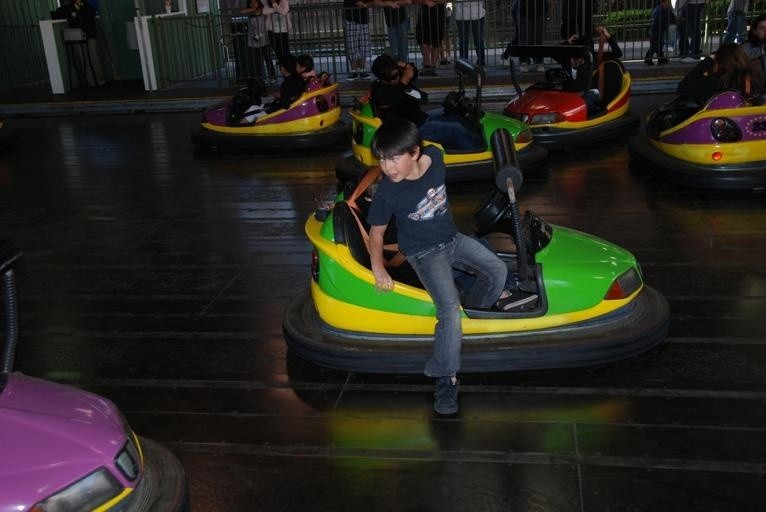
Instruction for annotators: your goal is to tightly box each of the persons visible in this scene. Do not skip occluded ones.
[342,0,373,81]
[241,54,316,126]
[240,0,277,83]
[512,1,766,112]
[374,0,412,63]
[52,1,107,88]
[440,3,452,65]
[454,0,486,73]
[262,0,292,76]
[366,116,509,414]
[370,53,473,149]
[413,0,445,76]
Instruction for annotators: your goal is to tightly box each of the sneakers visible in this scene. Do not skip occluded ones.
[497,293,539,311]
[434,377,460,414]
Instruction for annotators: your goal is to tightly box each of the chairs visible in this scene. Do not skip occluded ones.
[318,72,331,87]
[305,76,321,93]
[589,59,625,118]
[336,187,398,250]
[320,200,421,289]
